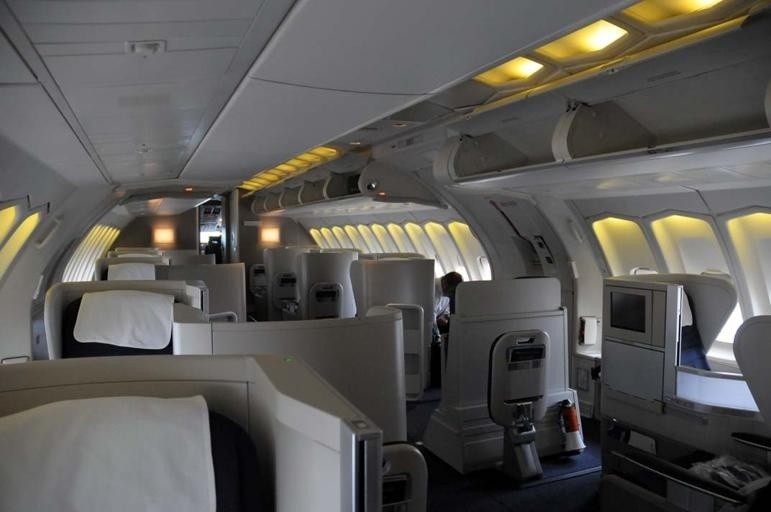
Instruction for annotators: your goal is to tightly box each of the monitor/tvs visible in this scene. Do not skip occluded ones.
[608,288,648,334]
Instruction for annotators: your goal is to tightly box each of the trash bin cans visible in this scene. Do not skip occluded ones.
[208,236,224,264]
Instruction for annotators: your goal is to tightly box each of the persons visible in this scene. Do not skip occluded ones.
[433,271,462,347]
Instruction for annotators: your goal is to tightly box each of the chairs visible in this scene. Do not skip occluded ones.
[423,277,586,479]
[599,272,771,473]
[0,244,435,511]
